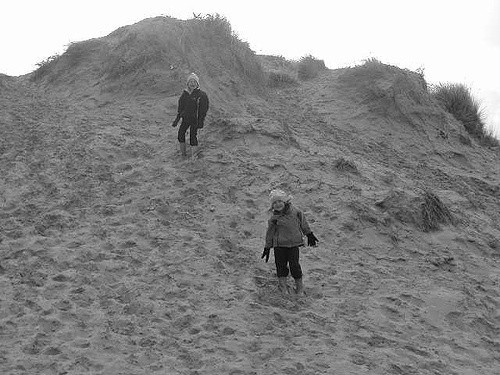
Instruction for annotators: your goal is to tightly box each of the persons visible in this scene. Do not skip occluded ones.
[261,188,319,296]
[172,73,209,162]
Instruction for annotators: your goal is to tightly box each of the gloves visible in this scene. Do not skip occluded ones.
[262,248,270,264]
[306,231,319,246]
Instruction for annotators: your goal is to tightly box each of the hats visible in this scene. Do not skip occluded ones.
[268,189,287,208]
[186,72,200,88]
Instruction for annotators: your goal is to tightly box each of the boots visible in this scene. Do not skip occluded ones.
[277,276,289,295]
[294,278,304,297]
[189,145,198,161]
[179,143,187,158]
